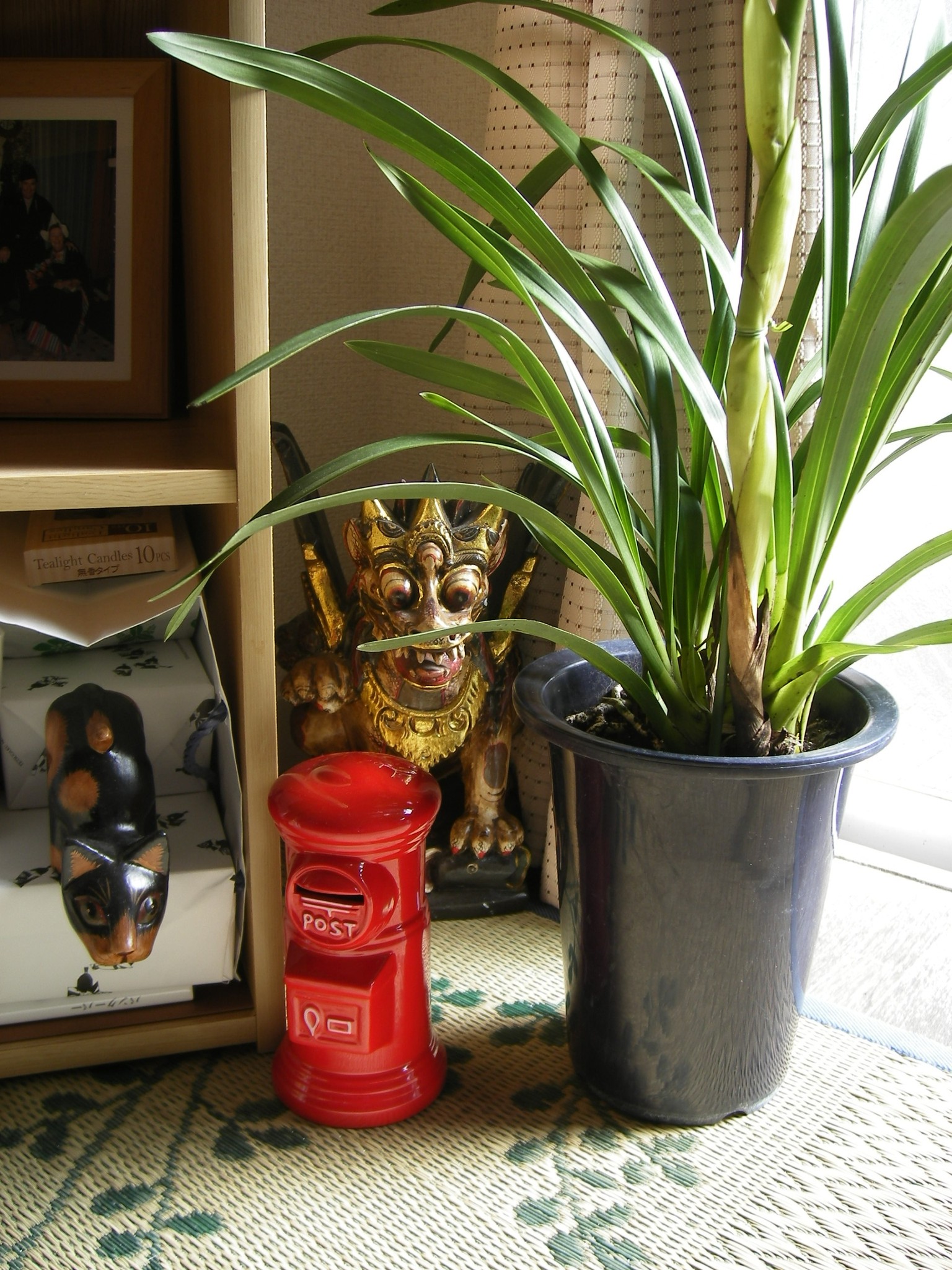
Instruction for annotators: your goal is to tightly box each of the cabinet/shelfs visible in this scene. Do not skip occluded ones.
[1,0,286,1083]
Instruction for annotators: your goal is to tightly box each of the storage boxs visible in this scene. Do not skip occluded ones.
[0,506,247,1030]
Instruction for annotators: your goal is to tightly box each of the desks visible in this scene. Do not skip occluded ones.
[1,915,952,1270]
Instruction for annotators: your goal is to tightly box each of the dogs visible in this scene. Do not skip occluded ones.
[41,683,173,967]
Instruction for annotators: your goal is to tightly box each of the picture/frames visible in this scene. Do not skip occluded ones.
[1,57,172,419]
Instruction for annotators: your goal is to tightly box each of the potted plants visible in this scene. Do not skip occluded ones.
[147,0,952,1123]
